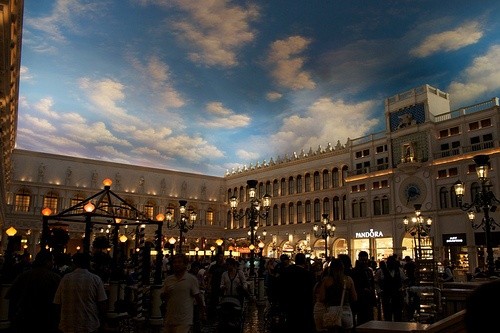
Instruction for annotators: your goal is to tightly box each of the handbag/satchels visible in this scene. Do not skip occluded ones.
[321,312,341,327]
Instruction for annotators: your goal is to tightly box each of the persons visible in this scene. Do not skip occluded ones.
[462,280,500,333]
[0,227,421,333]
[435,250,500,282]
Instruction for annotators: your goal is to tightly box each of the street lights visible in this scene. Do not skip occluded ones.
[403,203,433,260]
[5,177,166,288]
[454,154,500,277]
[210,237,224,262]
[166,199,197,258]
[230,178,273,272]
[315,212,336,260]
